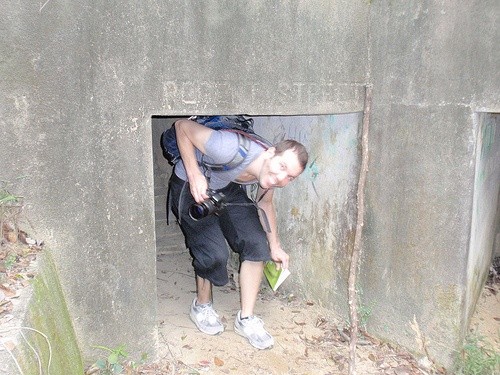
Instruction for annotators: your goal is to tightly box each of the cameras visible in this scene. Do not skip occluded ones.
[188,189,225,222]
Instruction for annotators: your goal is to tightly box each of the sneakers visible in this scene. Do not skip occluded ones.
[233,312,273,351]
[190,298,225,335]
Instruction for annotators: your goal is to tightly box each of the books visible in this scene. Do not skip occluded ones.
[262,259,291,293]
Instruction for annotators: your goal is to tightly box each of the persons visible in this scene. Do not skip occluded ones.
[166,118,308,350]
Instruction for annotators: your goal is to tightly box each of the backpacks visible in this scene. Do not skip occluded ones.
[160,115,254,165]
